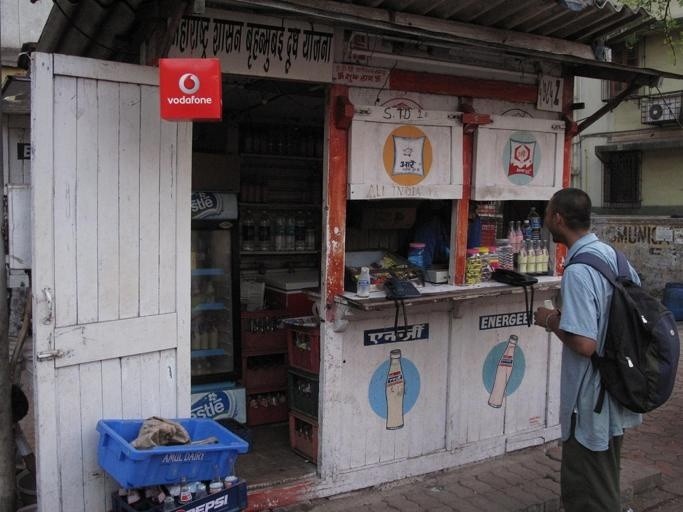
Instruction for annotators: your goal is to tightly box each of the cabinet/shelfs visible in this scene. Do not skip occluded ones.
[236,151,321,258]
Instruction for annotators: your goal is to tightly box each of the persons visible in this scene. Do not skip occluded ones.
[406,201,482,278]
[534,188,642,512]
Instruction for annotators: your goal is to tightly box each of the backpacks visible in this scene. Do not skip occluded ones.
[564,240,679,414]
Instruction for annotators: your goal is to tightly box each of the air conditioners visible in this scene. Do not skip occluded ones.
[646,98,682,123]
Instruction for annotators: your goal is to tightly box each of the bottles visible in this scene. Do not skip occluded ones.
[357,266,370,297]
[386,348,405,429]
[407,241,426,268]
[209,464,223,494]
[204,282,215,303]
[508,206,541,253]
[242,210,316,252]
[488,333,519,409]
[224,457,238,490]
[191,317,218,351]
[119,485,166,506]
[478,201,497,233]
[518,239,550,273]
[180,477,194,504]
[465,246,498,286]
[192,247,197,269]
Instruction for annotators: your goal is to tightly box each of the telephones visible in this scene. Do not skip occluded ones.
[492,269,538,286]
[384,278,421,299]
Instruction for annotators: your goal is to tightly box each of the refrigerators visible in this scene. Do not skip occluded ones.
[191,190,248,453]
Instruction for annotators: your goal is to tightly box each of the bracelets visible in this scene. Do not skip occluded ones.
[545,312,559,332]
